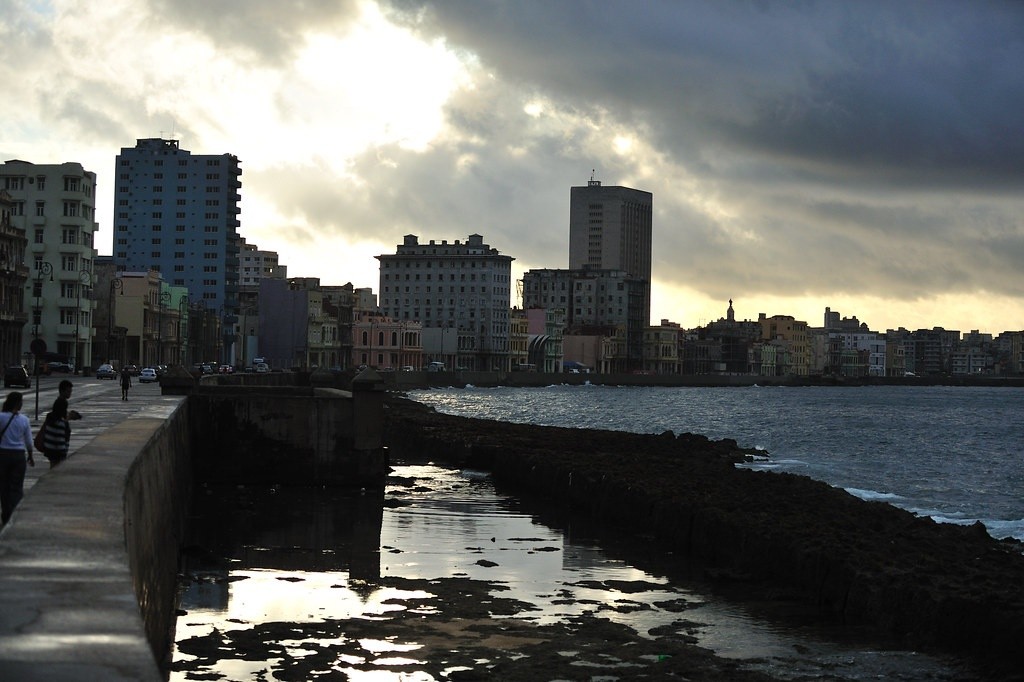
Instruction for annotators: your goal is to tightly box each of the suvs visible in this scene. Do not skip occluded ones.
[3,364,32,388]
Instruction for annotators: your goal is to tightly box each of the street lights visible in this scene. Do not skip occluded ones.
[176,294,192,365]
[368,320,381,366]
[157,279,172,365]
[195,298,209,362]
[440,323,450,363]
[73,269,93,376]
[34,259,56,422]
[216,305,231,366]
[595,333,607,373]
[107,278,126,364]
[400,320,409,367]
[554,332,564,372]
[304,313,318,372]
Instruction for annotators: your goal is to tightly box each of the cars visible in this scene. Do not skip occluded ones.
[244,357,273,373]
[120,363,139,378]
[95,365,118,380]
[43,362,76,377]
[138,357,234,384]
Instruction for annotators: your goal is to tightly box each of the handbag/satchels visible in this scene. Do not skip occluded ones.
[34,422,45,453]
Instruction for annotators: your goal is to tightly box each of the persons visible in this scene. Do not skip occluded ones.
[119,368,133,403]
[0,392,35,524]
[198,364,204,372]
[46,378,81,470]
[223,366,227,371]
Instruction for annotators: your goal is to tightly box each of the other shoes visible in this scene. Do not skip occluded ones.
[122,396,125,401]
[125,398,128,401]
